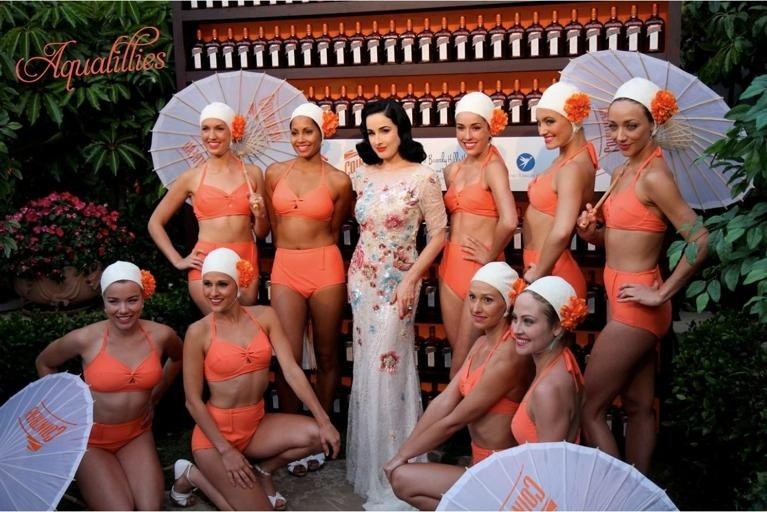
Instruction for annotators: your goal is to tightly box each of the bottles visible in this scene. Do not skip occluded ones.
[186,1,665,69]
[254,208,605,256]
[308,79,559,128]
[266,377,626,453]
[253,267,604,327]
[338,329,596,377]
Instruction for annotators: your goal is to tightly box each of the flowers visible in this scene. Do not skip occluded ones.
[141,269,157,299]
[232,114,246,143]
[322,110,340,139]
[564,91,592,122]
[491,109,509,136]
[236,258,256,289]
[508,278,529,306]
[650,89,679,123]
[0,192,138,283]
[560,295,590,332]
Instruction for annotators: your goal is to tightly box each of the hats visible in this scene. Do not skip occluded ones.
[609,76,662,137]
[536,81,584,134]
[288,103,325,142]
[201,246,242,296]
[200,101,235,136]
[524,274,579,322]
[100,261,144,297]
[454,90,495,130]
[469,261,520,317]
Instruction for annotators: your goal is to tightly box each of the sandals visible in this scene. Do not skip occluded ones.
[289,459,308,477]
[168,459,199,507]
[307,453,326,472]
[254,466,287,511]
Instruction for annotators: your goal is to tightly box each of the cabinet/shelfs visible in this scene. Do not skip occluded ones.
[172,2,682,447]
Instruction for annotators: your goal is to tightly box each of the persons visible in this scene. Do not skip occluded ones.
[437,90,518,380]
[509,275,585,447]
[520,82,599,305]
[349,98,447,512]
[146,102,264,313]
[577,76,709,474]
[384,262,530,512]
[263,102,352,421]
[34,260,182,512]
[172,246,340,511]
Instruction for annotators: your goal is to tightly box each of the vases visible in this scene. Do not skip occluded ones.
[13,261,102,308]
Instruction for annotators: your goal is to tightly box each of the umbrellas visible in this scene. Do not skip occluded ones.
[1,370,97,511]
[557,48,755,214]
[148,71,309,208]
[434,440,680,511]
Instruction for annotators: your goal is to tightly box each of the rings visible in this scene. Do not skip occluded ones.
[406,304,413,310]
